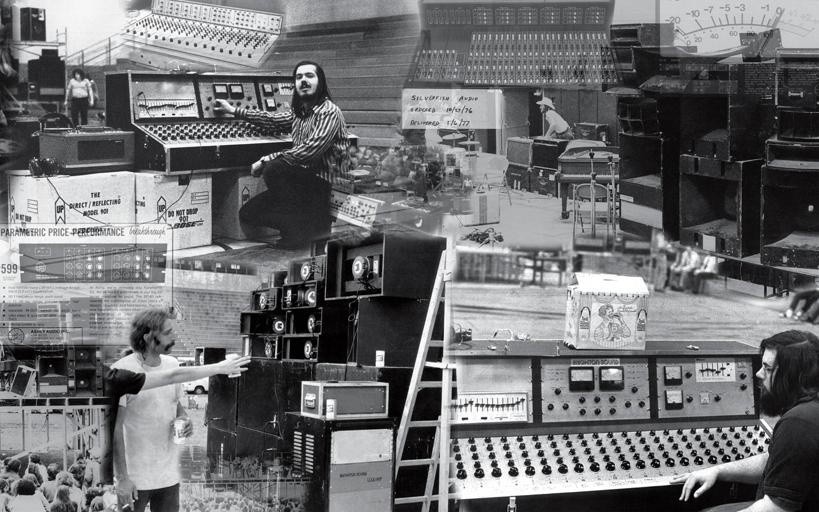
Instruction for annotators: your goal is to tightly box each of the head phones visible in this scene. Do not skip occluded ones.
[28,156,57,177]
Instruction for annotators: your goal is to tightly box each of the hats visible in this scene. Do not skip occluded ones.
[537,97,555,110]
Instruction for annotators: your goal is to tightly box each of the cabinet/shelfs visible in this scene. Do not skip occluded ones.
[203,234,446,511]
[599,19,819,272]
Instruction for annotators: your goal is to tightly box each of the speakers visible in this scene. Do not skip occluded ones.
[20,7,46,41]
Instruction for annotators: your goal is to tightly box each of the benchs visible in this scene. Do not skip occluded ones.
[263,16,422,148]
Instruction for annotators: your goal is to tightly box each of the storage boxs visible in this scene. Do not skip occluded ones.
[214,171,283,243]
[132,168,212,252]
[561,273,648,350]
[8,167,136,247]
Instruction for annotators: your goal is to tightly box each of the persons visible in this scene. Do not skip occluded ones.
[65,69,94,125]
[86,74,99,107]
[114,311,192,512]
[660,244,720,295]
[103,353,255,485]
[670,331,819,512]
[539,96,573,140]
[215,62,348,248]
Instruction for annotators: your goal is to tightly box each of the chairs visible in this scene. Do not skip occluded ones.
[459,151,515,211]
[572,179,615,249]
